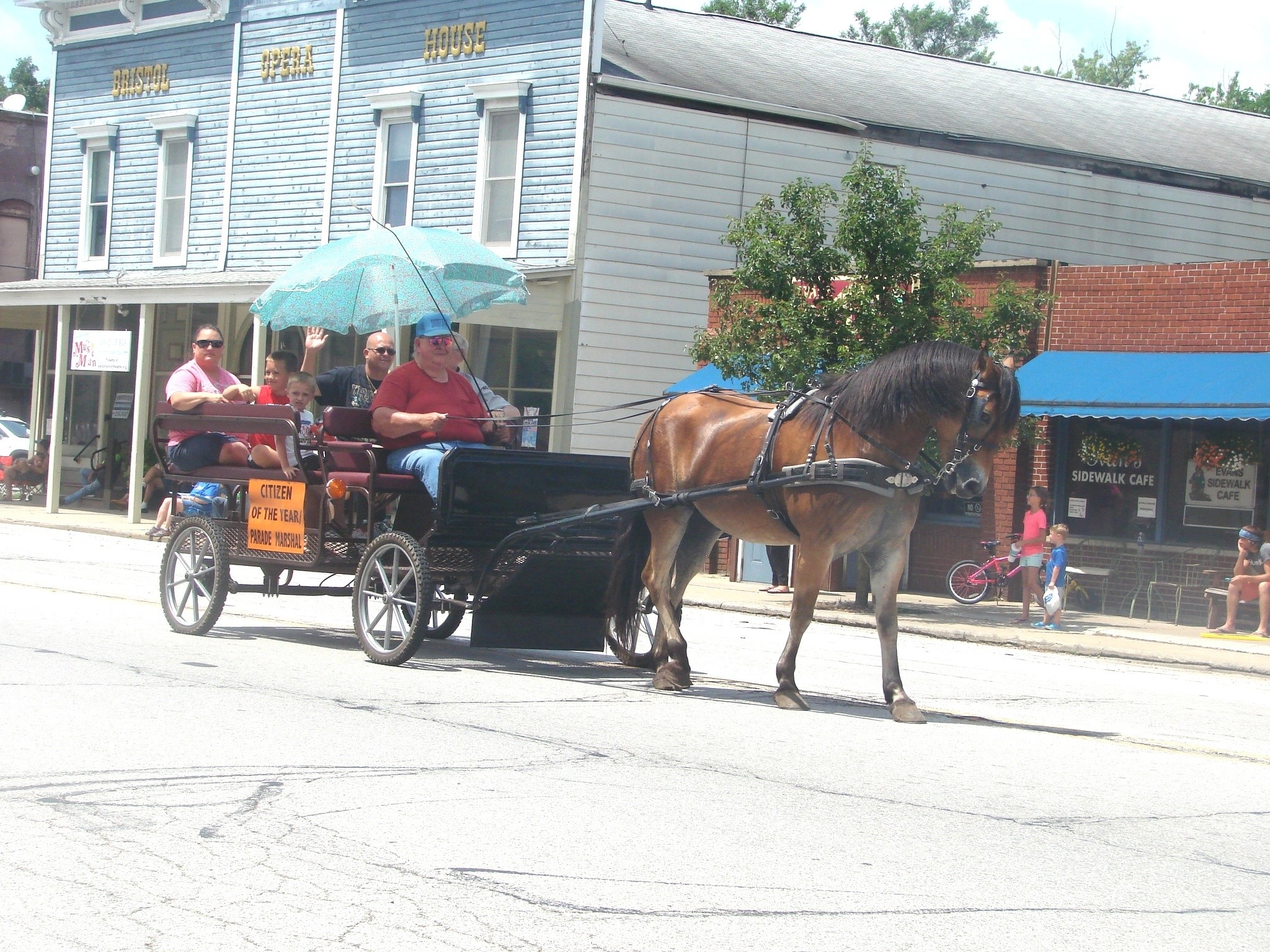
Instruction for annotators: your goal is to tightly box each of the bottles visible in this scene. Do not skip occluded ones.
[1137,532,1144,555]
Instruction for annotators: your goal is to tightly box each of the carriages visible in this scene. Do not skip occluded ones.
[152,339,1022,725]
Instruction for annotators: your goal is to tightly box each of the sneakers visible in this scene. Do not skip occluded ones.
[1032,621,1051,627]
[145,526,160,535]
[150,528,171,537]
[1045,622,1064,630]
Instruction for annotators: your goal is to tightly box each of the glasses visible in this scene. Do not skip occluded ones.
[420,336,452,346]
[368,347,396,356]
[1026,493,1037,498]
[192,340,224,348]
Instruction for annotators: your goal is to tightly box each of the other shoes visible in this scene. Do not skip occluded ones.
[59,495,66,507]
[1011,618,1029,624]
[1042,615,1054,622]
[111,499,128,510]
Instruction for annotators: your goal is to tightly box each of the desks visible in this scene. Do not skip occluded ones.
[1116,553,1170,619]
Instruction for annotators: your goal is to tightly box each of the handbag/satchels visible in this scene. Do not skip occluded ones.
[1043,585,1061,616]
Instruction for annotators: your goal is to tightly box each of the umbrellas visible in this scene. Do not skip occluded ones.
[249,223,530,366]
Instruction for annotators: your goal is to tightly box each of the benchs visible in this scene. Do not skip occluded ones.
[317,405,423,493]
[150,401,324,490]
[1202,569,1260,630]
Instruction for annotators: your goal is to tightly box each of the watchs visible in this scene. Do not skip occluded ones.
[495,409,505,417]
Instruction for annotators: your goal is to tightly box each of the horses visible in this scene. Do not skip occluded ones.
[612,341,1020,724]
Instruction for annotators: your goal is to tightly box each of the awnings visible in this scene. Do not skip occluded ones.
[663,354,873,400]
[1016,351,1270,421]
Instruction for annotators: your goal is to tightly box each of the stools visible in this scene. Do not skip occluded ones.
[11,481,45,501]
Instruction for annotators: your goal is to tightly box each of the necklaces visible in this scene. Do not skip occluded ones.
[366,375,377,397]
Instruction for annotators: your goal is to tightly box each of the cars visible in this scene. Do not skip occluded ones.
[0,416,37,467]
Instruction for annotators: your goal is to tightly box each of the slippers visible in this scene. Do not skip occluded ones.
[1249,632,1269,638]
[1209,627,1237,634]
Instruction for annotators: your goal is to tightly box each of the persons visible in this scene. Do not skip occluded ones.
[59,443,121,506]
[368,313,510,505]
[1189,465,1205,497]
[445,331,521,423]
[1070,462,1123,539]
[274,371,351,538]
[1031,523,1069,630]
[1011,486,1050,624]
[145,482,220,537]
[0,439,50,501]
[223,351,298,468]
[759,545,789,593]
[166,324,250,471]
[300,327,396,537]
[110,443,193,513]
[1209,526,1270,637]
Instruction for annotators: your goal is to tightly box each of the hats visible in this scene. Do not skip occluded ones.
[416,313,451,337]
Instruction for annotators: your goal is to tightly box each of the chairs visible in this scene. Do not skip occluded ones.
[0,456,13,497]
[1063,537,1126,614]
[1147,544,1221,626]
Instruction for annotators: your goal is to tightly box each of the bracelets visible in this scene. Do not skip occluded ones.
[1239,575,1241,584]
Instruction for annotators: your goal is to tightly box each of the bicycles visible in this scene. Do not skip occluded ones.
[946,533,1047,608]
